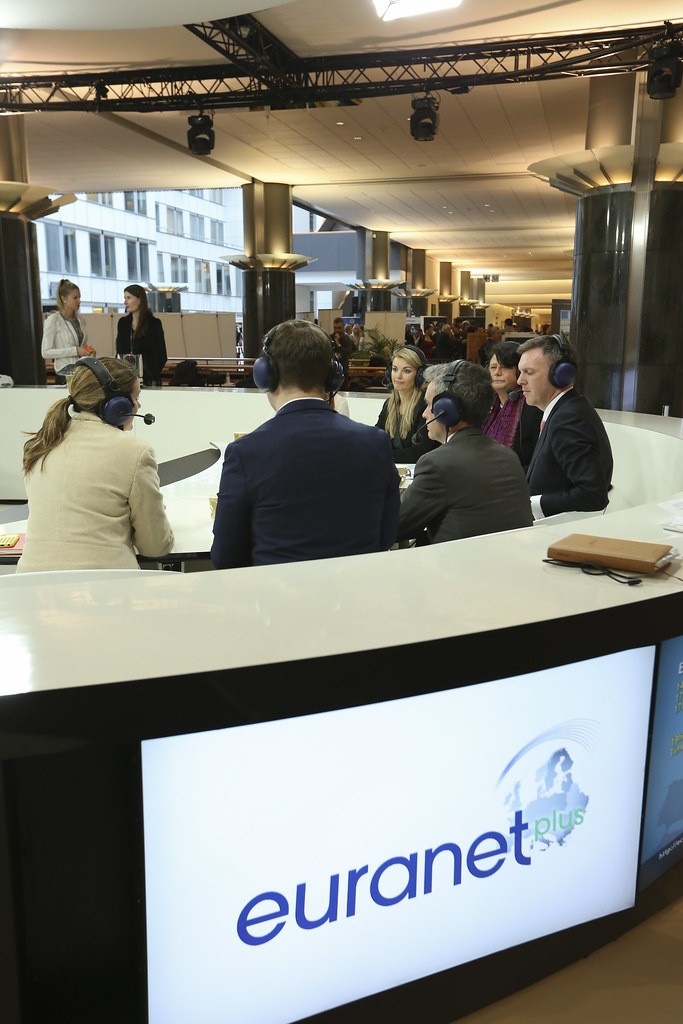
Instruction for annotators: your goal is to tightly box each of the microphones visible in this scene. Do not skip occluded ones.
[382,377,394,390]
[119,411,155,425]
[412,410,446,445]
[509,390,522,402]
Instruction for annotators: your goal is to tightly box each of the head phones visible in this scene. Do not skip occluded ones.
[385,345,427,387]
[550,333,578,388]
[431,358,468,427]
[76,356,133,426]
[253,321,344,393]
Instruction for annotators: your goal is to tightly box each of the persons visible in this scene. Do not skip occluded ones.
[115,284,167,386]
[16,357,175,574]
[234,316,613,545]
[42,279,94,386]
[208,319,400,566]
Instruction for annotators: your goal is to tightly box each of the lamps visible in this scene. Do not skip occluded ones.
[410,90,440,141]
[187,108,215,155]
[647,33,683,99]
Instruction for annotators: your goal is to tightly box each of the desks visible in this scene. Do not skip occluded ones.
[0,441,416,572]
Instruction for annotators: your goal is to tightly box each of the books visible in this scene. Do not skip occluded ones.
[0,533,26,555]
